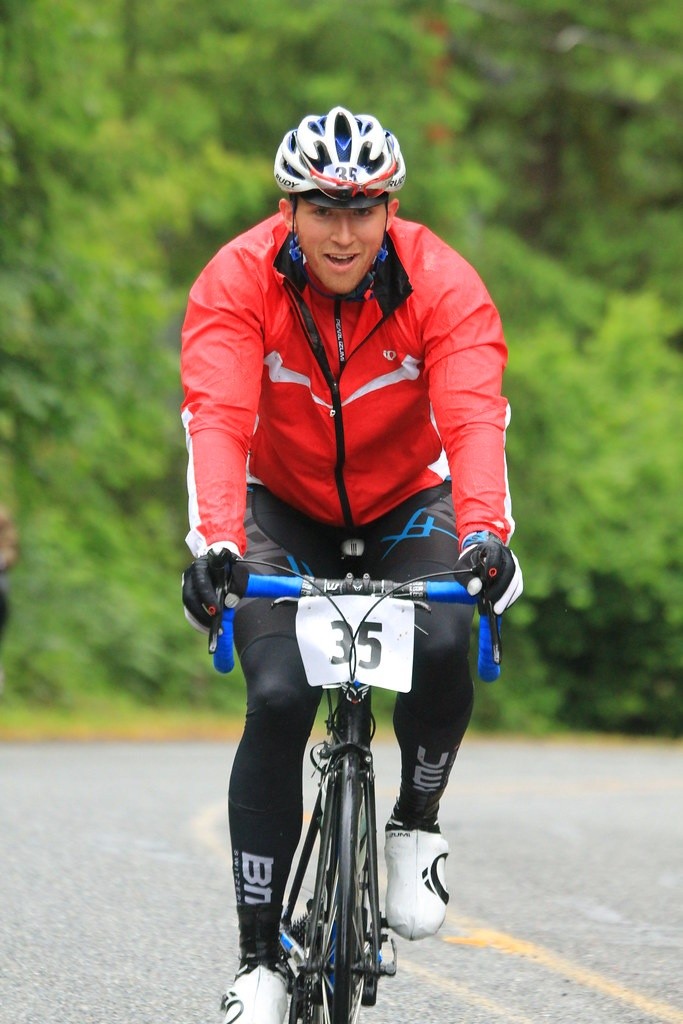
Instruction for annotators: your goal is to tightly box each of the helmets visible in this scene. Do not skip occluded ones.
[274,105,407,210]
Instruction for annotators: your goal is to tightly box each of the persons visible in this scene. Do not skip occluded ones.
[176,107,524,1023]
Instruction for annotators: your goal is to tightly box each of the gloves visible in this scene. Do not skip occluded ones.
[452,542,524,616]
[181,550,250,636]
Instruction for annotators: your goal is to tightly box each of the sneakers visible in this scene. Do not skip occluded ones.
[223,964,288,1024]
[385,815,449,941]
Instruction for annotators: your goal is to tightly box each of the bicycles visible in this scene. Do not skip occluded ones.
[203,537,505,1024]
